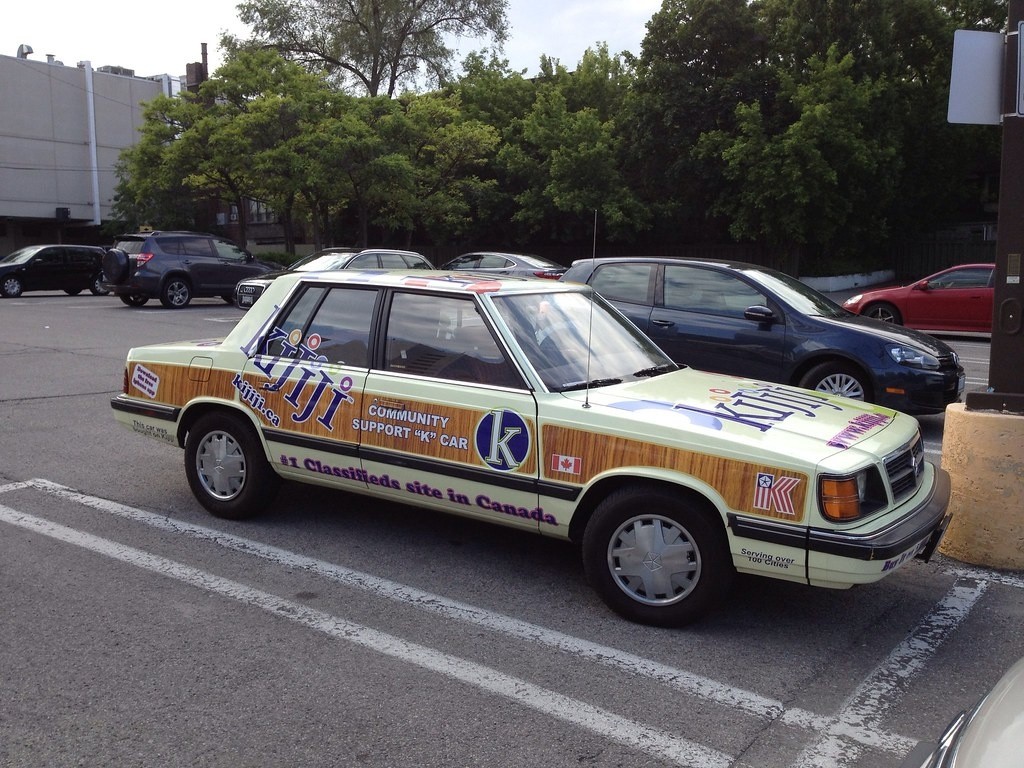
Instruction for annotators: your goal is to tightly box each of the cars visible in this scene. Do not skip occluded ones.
[230,246,437,312]
[109,268,953,629]
[436,252,567,281]
[841,263,996,332]
[0,244,112,299]
[561,256,966,420]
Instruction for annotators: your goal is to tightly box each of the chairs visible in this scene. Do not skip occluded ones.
[698,271,725,307]
[668,275,696,307]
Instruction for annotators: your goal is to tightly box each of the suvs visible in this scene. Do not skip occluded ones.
[102,229,274,310]
